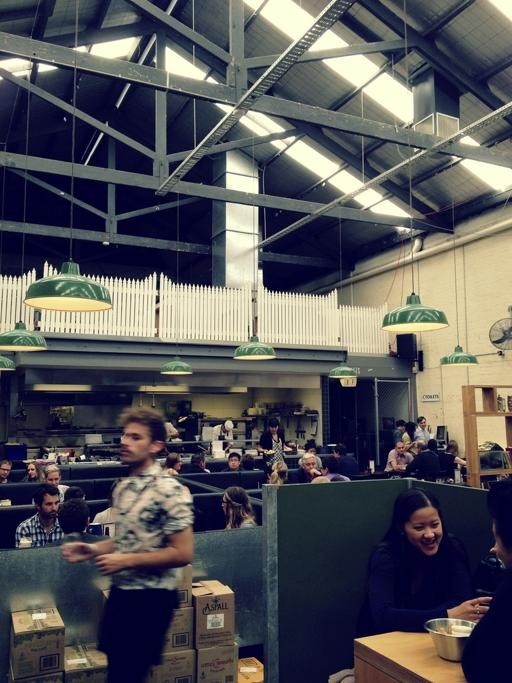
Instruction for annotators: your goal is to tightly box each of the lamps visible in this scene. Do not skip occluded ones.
[327,205,358,376]
[380,1,449,334]
[234,111,278,361]
[439,156,478,367]
[23,1,111,312]
[0,354,16,373]
[159,194,193,375]
[1,62,47,352]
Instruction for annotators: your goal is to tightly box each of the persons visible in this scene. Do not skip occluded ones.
[462,477,512,683]
[60,408,195,682]
[342,487,494,668]
[384,416,466,482]
[0,406,358,548]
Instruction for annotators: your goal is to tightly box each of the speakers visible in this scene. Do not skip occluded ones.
[395,333,417,363]
[417,351,425,372]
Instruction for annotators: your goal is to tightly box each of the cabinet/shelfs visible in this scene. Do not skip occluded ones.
[461,384,512,490]
[201,415,256,446]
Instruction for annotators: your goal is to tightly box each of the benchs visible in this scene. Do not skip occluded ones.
[262,478,490,683]
[0,444,390,531]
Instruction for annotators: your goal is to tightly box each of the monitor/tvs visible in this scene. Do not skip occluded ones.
[436,425,450,445]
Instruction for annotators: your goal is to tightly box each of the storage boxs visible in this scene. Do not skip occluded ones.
[238,656,265,683]
[8,564,239,683]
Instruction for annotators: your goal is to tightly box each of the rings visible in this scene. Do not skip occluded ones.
[475,605,480,615]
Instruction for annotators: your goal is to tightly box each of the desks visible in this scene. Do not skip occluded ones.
[354,630,467,683]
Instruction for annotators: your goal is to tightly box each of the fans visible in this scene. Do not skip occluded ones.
[489,317,512,351]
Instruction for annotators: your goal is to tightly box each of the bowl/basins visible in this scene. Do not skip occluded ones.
[424,618,477,662]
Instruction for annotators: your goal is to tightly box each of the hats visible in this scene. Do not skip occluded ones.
[224,420,233,431]
